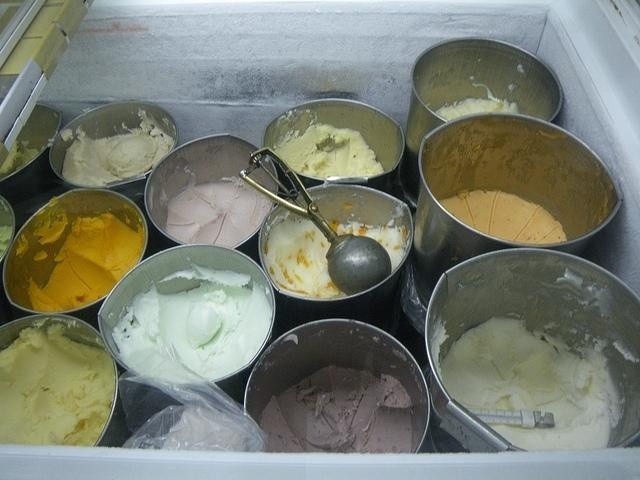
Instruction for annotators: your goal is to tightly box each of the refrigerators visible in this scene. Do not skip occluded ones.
[1,0,640,479]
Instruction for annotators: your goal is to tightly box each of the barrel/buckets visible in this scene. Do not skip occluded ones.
[2,103,62,232]
[97,244,277,404]
[399,35,564,207]
[413,112,623,307]
[258,185,413,336]
[424,248,639,451]
[243,318,430,453]
[2,189,148,329]
[261,98,405,196]
[143,132,279,264]
[48,100,180,215]
[1,314,129,447]
[1,194,16,261]
